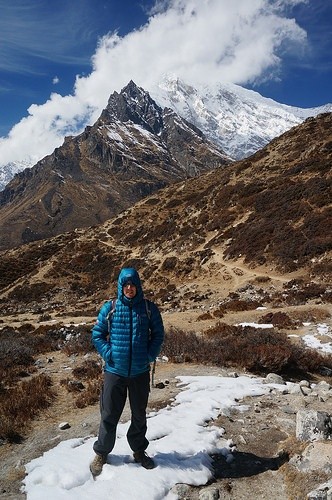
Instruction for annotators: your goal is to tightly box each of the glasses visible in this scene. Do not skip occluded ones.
[123,282,135,287]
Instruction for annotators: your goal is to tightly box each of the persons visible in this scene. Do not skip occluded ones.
[87,268,165,478]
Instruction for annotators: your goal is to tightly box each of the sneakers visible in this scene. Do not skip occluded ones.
[90,453,106,475]
[133,449,154,469]
